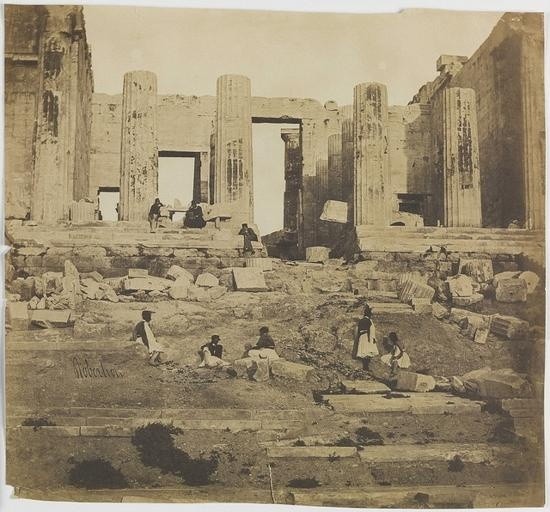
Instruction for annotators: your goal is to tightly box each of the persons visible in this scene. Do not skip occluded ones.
[23,206,31,220]
[148,198,162,233]
[115,203,118,213]
[382,332,411,374]
[285,224,298,261]
[180,199,207,229]
[247,326,282,360]
[238,223,258,256]
[279,224,290,260]
[198,334,232,369]
[135,310,165,366]
[99,210,102,220]
[356,308,379,372]
